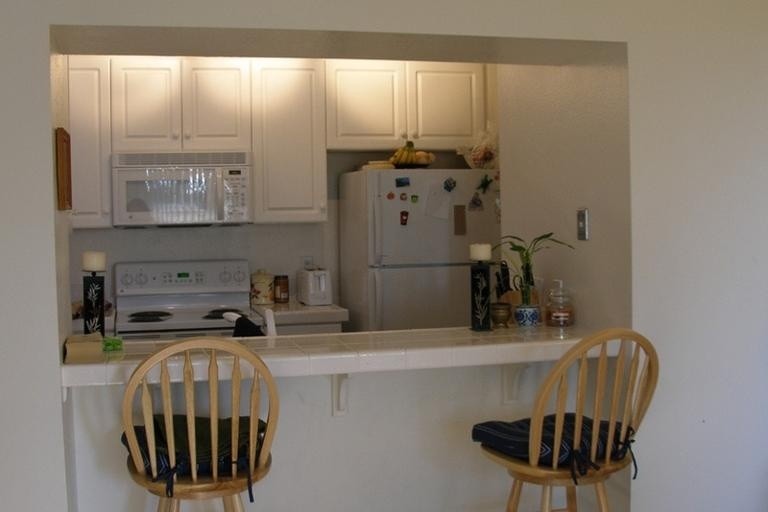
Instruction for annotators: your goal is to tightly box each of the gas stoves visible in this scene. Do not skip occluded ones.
[113,305,264,334]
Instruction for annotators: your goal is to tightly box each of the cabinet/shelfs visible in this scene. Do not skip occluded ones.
[113,53,252,154]
[67,53,113,230]
[252,57,328,226]
[326,58,485,152]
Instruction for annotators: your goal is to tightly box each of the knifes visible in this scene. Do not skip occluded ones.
[495,262,512,297]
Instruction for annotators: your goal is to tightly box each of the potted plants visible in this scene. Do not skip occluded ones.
[492,229,578,327]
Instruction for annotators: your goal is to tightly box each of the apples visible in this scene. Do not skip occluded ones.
[416,151,431,164]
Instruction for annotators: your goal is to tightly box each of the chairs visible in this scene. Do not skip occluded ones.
[121,334,281,512]
[481,326,660,512]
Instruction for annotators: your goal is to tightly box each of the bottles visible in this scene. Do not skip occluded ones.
[250,267,274,305]
[546,287,575,325]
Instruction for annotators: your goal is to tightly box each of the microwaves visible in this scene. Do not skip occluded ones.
[110,162,253,229]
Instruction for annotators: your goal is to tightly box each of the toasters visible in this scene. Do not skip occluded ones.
[296,267,332,307]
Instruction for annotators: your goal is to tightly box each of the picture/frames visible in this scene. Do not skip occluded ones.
[53,124,73,211]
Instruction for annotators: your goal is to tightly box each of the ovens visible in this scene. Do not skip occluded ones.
[115,328,261,344]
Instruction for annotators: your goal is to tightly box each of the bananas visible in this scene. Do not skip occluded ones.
[389,141,416,166]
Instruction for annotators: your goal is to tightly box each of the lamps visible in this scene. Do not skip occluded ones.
[469,242,494,332]
[80,250,107,339]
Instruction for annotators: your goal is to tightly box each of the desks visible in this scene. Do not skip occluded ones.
[62,324,633,419]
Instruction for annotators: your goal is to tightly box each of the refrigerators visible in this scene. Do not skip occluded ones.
[337,166,501,331]
